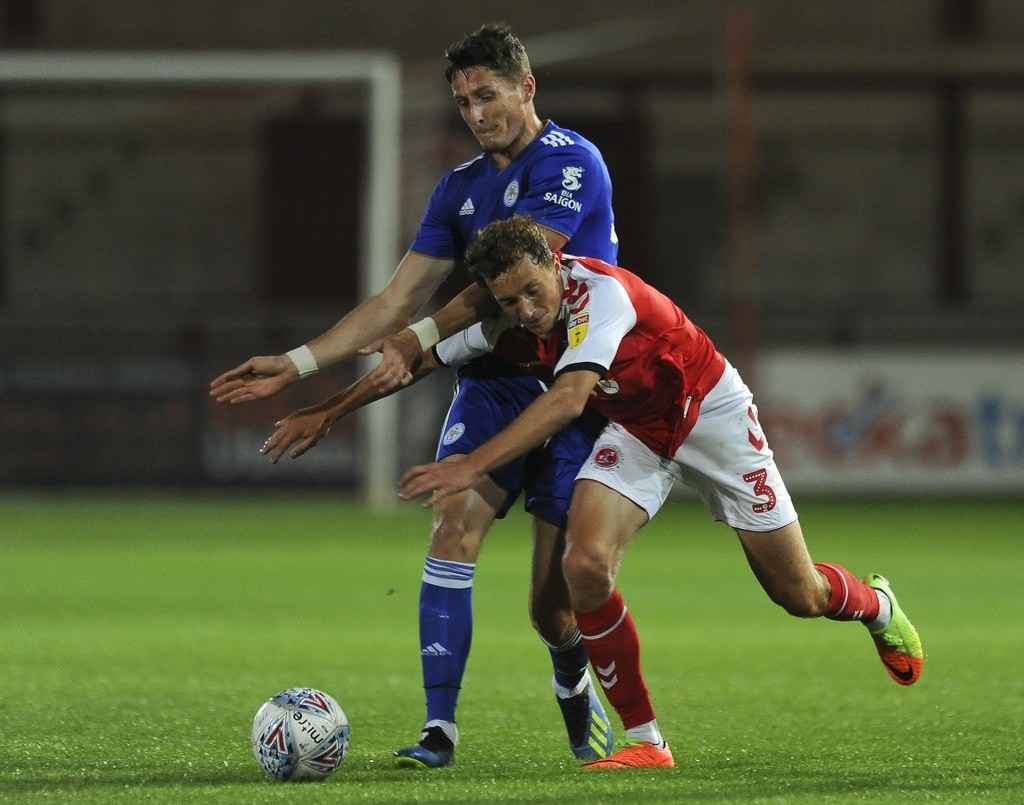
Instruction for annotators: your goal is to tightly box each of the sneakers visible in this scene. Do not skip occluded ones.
[552,666,613,760]
[393,726,455,770]
[580,736,674,770]
[859,573,924,685]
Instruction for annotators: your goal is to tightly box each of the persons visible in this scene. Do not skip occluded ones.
[209,24,618,768]
[261,219,923,768]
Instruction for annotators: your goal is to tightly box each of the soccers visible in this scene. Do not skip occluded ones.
[251,687,351,783]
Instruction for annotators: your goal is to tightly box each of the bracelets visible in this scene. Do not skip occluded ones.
[286,345,318,379]
[407,316,441,353]
